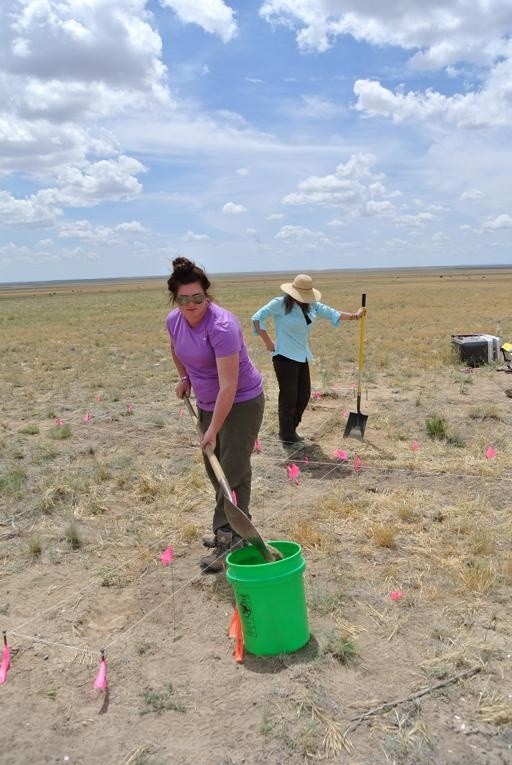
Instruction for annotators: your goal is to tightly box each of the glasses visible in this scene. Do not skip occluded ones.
[176,294,203,307]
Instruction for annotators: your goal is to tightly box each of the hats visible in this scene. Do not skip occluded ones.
[280,273,321,303]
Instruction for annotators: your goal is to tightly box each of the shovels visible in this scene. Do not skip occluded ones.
[342,294,369,441]
[183,391,273,563]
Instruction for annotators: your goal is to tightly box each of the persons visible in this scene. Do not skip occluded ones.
[165,257,264,572]
[251,275,367,443]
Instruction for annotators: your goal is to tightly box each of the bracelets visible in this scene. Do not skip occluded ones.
[350,314,358,320]
[181,376,188,381]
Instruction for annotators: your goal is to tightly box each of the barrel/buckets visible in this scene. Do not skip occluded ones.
[225,541,311,658]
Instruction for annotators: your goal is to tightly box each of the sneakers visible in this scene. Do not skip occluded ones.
[282,435,312,442]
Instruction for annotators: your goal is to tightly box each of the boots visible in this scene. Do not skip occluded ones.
[200,529,249,572]
